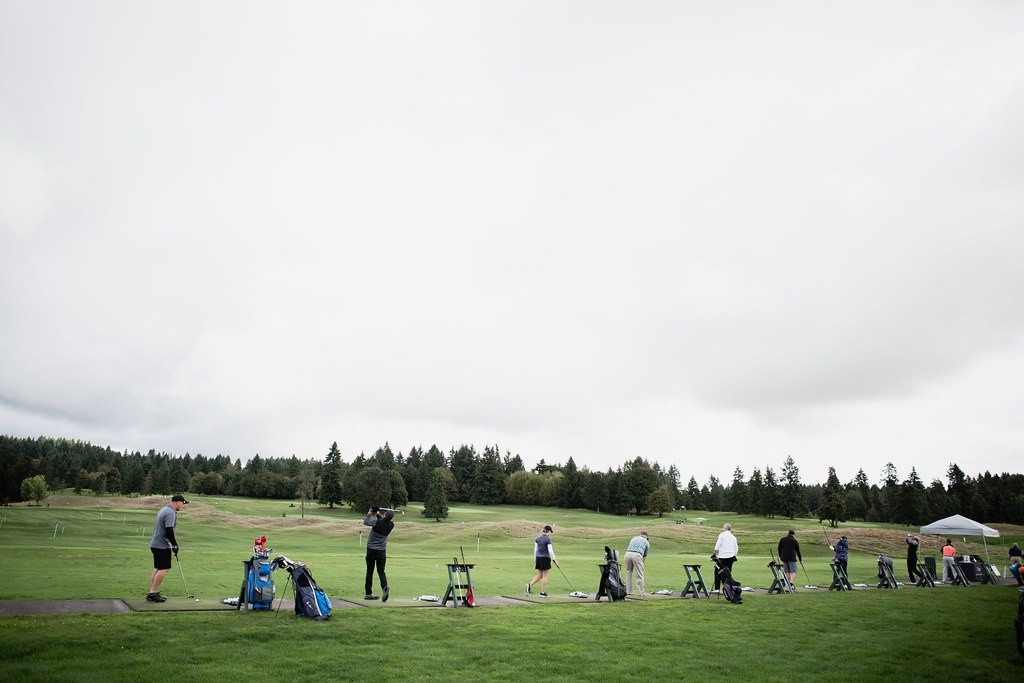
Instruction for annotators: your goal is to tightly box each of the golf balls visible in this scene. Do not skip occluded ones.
[196,599,199,602]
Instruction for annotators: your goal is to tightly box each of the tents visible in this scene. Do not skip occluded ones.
[920,514,1014,579]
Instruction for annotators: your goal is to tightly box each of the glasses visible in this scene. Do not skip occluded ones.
[912,538,914,539]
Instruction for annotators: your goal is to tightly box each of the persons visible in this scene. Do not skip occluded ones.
[829,536,848,588]
[711,523,739,593]
[940,538,962,585]
[526,525,558,597]
[1009,543,1022,566]
[364,504,395,603]
[905,534,925,585]
[624,532,650,597]
[778,530,803,589]
[146,495,185,603]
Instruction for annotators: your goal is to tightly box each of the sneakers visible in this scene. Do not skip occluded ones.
[382,586,389,602]
[539,592,550,598]
[364,594,379,600]
[146,593,167,602]
[524,583,531,597]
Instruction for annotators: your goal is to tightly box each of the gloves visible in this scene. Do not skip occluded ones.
[555,560,558,566]
[829,544,834,550]
[172,545,179,556]
[369,505,379,514]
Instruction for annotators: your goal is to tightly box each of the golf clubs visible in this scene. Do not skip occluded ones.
[452,544,472,607]
[800,562,812,587]
[252,545,274,557]
[614,549,620,560]
[604,552,608,564]
[379,508,405,516]
[711,559,720,570]
[553,560,578,595]
[822,522,831,545]
[645,577,655,594]
[295,561,305,567]
[175,554,194,598]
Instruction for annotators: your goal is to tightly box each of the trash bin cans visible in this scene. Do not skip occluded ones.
[924,557,937,579]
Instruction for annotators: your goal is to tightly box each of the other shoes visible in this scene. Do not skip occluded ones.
[711,590,720,593]
[911,583,917,584]
[791,586,796,591]
[922,579,925,583]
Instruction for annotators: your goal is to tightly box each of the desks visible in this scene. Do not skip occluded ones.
[958,562,989,584]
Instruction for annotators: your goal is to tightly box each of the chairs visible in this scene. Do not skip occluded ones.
[922,557,937,579]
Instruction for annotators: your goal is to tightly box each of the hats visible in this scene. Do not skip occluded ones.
[641,531,648,536]
[385,510,395,519]
[543,525,553,533]
[841,536,847,540]
[947,539,951,543]
[1013,543,1018,547]
[172,495,189,504]
